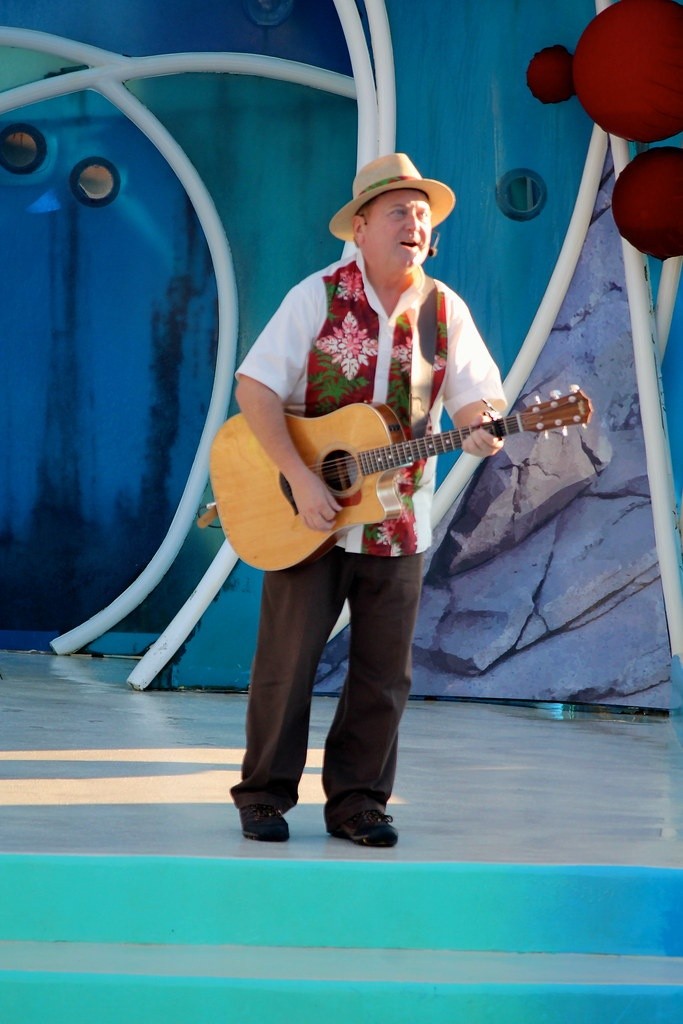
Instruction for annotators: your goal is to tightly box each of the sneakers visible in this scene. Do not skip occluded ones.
[331,810,398,847]
[240,803,289,842]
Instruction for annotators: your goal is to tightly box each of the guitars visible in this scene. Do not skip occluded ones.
[208,383,595,573]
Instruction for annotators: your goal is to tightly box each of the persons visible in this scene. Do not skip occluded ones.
[235,152,507,848]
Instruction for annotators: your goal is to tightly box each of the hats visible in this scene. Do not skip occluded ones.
[328,154,456,241]
